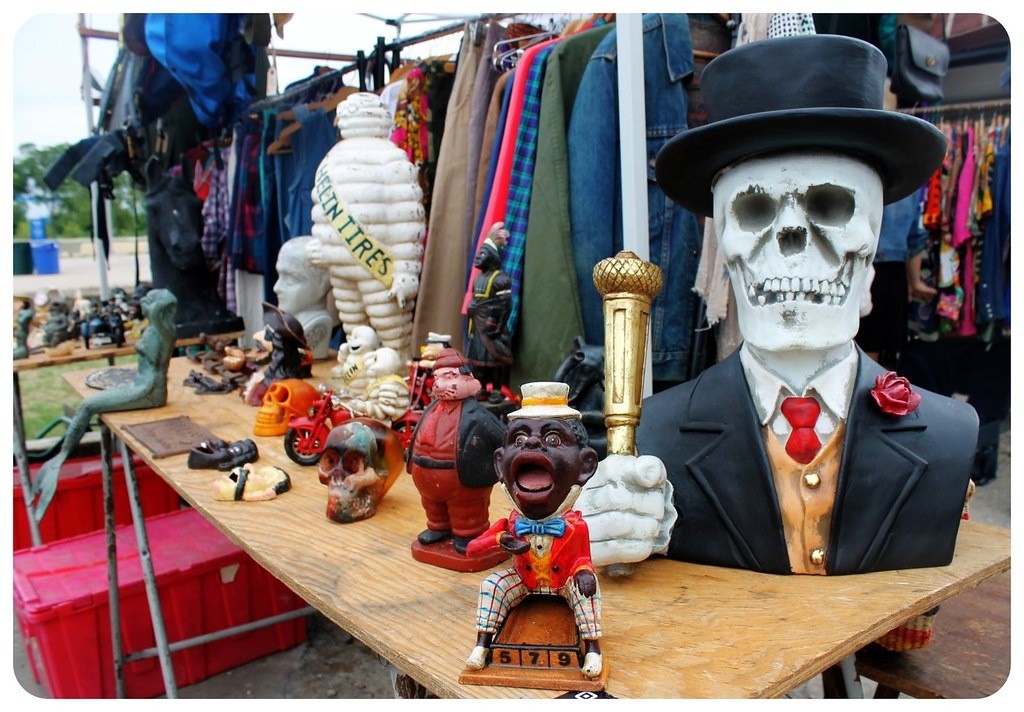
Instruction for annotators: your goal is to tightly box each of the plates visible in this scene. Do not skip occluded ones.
[86,369,134,389]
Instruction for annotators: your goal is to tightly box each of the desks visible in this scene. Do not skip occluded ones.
[63,355,1011,698]
[13,328,249,548]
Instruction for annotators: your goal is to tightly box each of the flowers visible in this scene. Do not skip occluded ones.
[870,370,922,419]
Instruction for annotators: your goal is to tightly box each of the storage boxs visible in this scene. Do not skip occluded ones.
[13,452,179,551]
[13,511,305,699]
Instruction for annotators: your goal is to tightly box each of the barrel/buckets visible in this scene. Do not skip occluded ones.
[30,241,59,274]
[13,243,32,273]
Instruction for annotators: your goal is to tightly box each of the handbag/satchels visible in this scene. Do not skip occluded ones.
[891,23,949,104]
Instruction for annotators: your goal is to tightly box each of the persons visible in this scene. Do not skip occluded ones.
[337,326,377,398]
[571,35,979,575]
[26,289,178,524]
[273,237,332,359]
[466,221,514,404]
[407,349,508,553]
[197,334,246,372]
[304,93,425,376]
[223,302,307,403]
[13,286,146,360]
[856,72,938,362]
[406,333,452,410]
[352,348,410,425]
[464,382,605,677]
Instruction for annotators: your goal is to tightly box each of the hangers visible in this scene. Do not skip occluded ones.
[128,13,1011,156]
